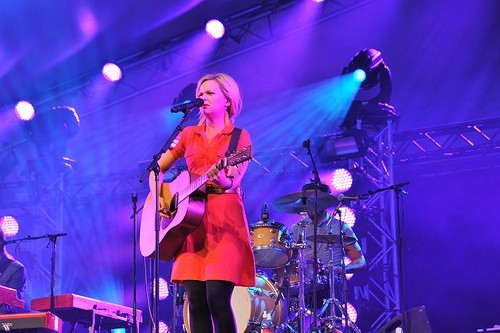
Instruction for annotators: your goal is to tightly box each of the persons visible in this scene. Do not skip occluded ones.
[0,228,28,314]
[149,72,255,333]
[289,184,366,333]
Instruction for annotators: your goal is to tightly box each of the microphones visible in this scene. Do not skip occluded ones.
[263,202,269,221]
[336,193,361,201]
[302,139,311,147]
[326,202,342,226]
[170,98,204,113]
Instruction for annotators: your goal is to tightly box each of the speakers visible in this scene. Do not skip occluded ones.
[378,305,433,333]
[0,312,63,333]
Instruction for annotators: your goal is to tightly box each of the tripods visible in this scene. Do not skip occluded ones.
[274,145,362,333]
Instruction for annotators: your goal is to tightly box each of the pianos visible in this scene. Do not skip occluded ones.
[0,284,142,333]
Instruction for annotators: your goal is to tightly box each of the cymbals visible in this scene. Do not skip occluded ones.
[305,234,356,244]
[273,192,337,214]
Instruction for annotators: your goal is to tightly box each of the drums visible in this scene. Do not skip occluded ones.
[272,259,329,296]
[248,222,291,269]
[183,274,284,333]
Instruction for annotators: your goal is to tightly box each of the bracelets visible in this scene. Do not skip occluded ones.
[221,178,233,191]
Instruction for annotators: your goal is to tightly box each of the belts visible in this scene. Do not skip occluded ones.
[205,185,241,194]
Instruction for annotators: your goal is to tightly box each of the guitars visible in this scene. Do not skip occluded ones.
[139,144,254,262]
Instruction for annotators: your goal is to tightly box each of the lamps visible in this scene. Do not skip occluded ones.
[338,48,398,130]
